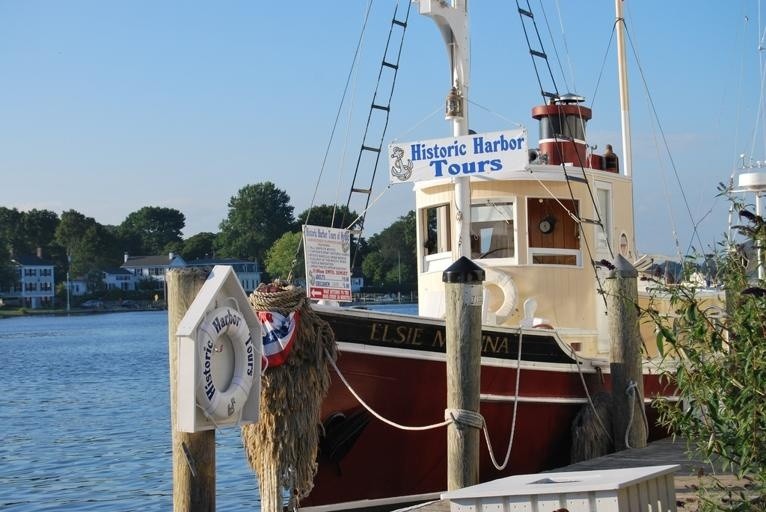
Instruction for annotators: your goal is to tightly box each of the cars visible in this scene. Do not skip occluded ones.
[112,300,138,307]
[82,300,105,308]
[358,294,376,301]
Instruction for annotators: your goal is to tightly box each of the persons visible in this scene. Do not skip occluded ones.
[154,293,160,302]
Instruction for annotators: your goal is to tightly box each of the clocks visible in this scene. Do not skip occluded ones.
[539,220,554,233]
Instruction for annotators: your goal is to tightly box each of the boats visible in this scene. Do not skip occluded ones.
[234,0,728,510]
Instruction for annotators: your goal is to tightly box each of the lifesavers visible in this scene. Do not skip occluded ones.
[197,308,255,418]
